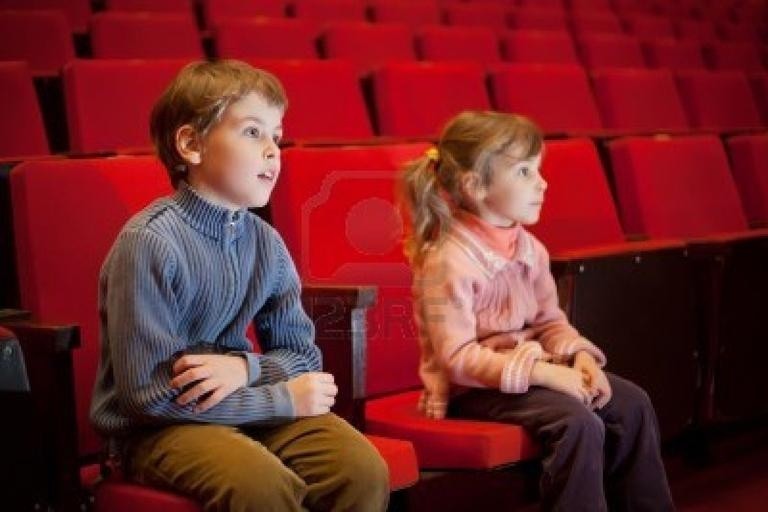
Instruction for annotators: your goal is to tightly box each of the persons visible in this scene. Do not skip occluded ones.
[398,110,675,510]
[91,59,391,511]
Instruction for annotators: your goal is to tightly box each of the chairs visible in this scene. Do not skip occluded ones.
[727,131,764,239]
[604,130,761,434]
[525,133,701,432]
[9,155,424,510]
[3,4,763,180]
[273,143,582,510]
